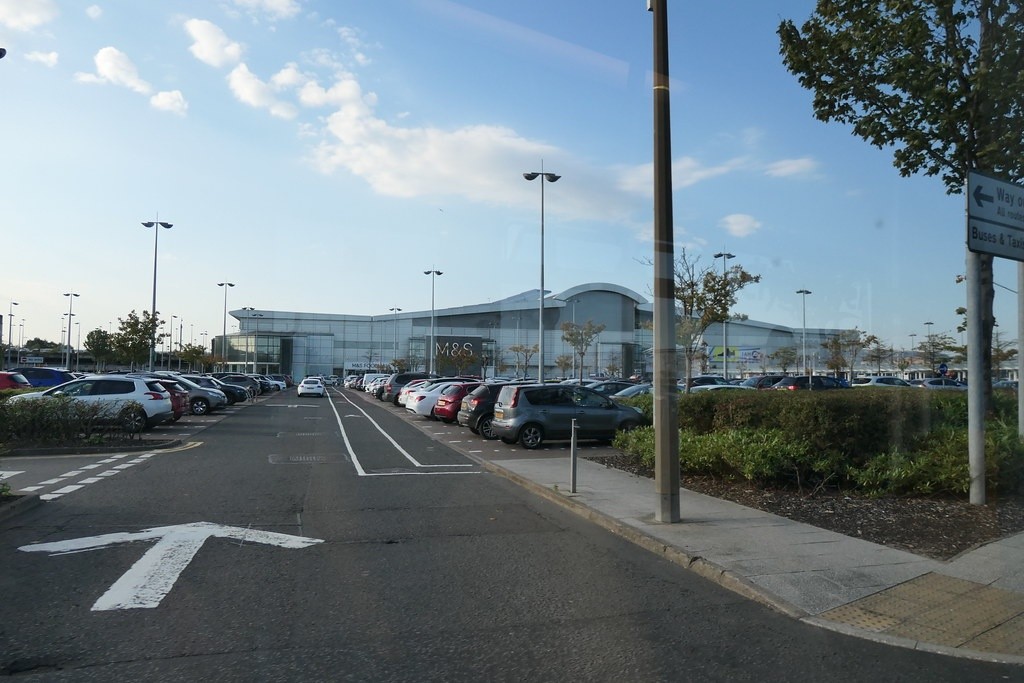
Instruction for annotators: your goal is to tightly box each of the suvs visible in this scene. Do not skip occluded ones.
[4,372,175,434]
[4,366,294,426]
[490,383,651,450]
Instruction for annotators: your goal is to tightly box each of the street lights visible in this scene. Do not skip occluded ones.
[924,322,934,369]
[796,287,813,374]
[423,264,444,374]
[8,302,19,368]
[909,334,917,365]
[18,325,23,350]
[252,313,264,373]
[63,292,80,371]
[140,213,174,371]
[523,158,562,383]
[389,305,402,374]
[713,243,736,380]
[241,307,255,374]
[22,319,26,346]
[218,282,236,372]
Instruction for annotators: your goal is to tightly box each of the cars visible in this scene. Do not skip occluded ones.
[0,371,34,391]
[297,378,326,398]
[304,373,1017,442]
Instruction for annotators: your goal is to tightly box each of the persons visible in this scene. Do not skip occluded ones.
[246,386,256,399]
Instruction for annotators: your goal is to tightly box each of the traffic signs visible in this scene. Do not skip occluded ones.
[966,170,1024,263]
